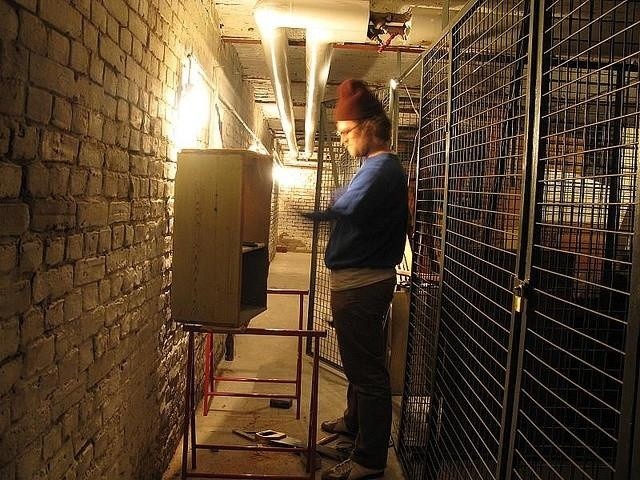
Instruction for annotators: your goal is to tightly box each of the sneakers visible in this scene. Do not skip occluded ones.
[321,458,384,480]
[322,417,358,436]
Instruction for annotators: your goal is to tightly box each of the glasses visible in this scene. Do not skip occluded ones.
[336,121,366,137]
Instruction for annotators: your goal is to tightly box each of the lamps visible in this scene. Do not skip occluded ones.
[390,78,402,90]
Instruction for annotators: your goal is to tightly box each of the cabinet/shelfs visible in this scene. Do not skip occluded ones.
[183,287,330,480]
[168,145,275,329]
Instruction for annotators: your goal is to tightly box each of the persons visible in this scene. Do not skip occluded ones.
[302,77,409,480]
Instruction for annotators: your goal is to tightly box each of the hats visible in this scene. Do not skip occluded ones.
[334,79,381,121]
